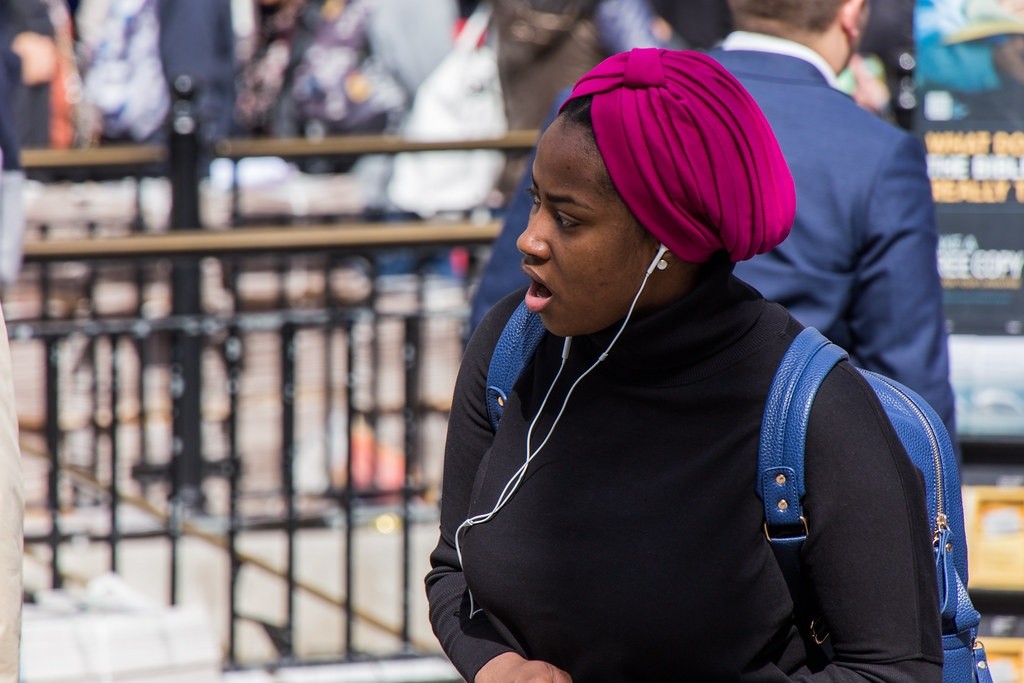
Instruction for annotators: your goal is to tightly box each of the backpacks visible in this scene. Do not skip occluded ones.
[483,299,994,683]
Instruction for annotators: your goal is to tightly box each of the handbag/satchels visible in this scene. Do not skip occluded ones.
[385,3,508,214]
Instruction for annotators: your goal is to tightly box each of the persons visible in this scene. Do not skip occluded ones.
[421,50,942,683]
[0,0,734,288]
[471,0,958,471]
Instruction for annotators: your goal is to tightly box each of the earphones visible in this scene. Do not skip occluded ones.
[647,244,669,276]
